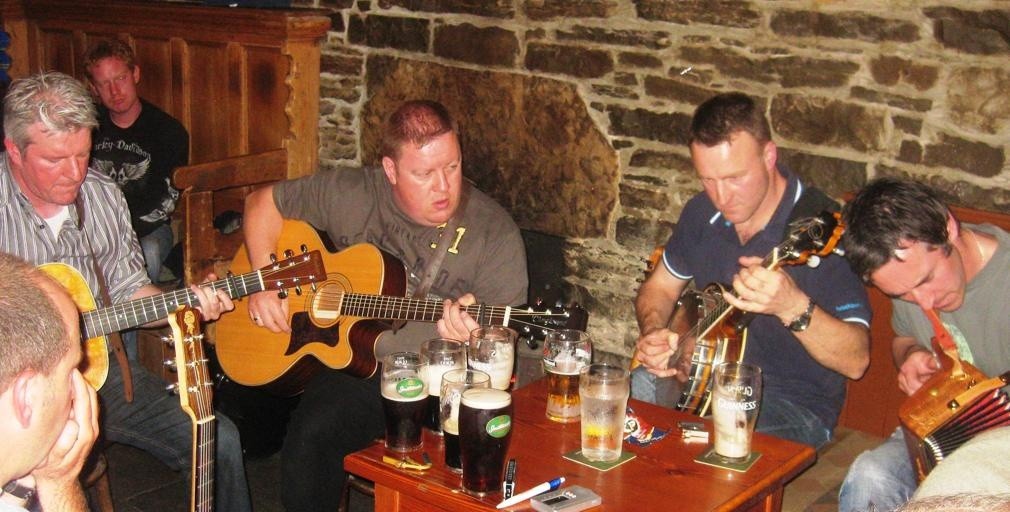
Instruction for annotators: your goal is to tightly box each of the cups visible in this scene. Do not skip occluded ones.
[438,368,493,476]
[711,363,764,464]
[457,389,515,496]
[467,326,515,392]
[577,362,631,466]
[418,337,470,436]
[379,351,430,453]
[543,329,595,426]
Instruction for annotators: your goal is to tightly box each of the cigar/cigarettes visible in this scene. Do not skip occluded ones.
[705,209,727,227]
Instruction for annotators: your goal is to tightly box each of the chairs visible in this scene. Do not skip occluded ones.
[81,327,190,511]
[173,149,289,291]
[826,193,1010,438]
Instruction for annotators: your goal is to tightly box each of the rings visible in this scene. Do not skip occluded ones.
[255,314,259,323]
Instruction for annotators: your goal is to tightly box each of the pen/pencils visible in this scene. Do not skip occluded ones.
[496,476,565,509]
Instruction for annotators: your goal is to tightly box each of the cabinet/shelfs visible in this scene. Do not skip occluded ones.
[1,2,332,220]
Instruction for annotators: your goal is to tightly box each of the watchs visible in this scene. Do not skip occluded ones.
[784,295,815,332]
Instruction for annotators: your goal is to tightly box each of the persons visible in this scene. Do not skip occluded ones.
[0,254,102,512]
[840,175,1010,510]
[0,67,251,512]
[245,99,529,512]
[80,41,189,290]
[633,91,875,458]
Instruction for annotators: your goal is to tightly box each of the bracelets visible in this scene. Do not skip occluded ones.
[3,478,38,500]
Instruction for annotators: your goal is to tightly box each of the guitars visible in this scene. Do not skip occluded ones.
[31,242,327,393]
[628,207,848,416]
[211,220,588,396]
